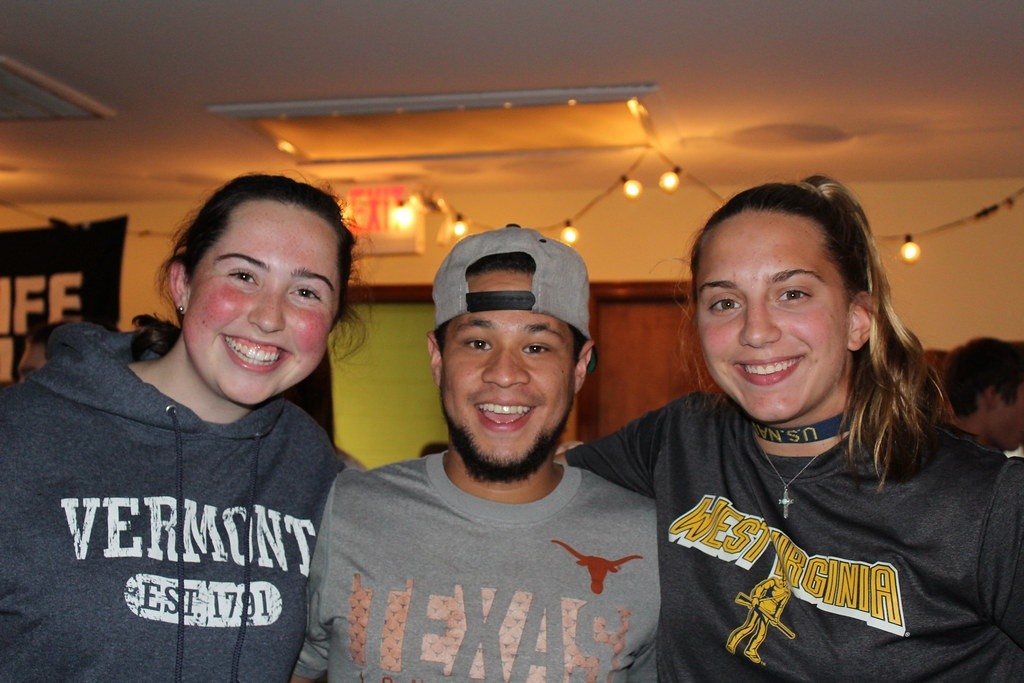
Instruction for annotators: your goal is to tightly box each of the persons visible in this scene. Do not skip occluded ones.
[17,320,83,386]
[0,172,368,683]
[554,172,1024,683]
[289,224,655,683]
[936,337,1024,452]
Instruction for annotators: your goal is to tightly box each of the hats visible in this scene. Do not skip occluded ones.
[432,222,597,372]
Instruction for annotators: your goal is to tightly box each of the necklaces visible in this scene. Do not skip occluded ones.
[758,442,825,518]
